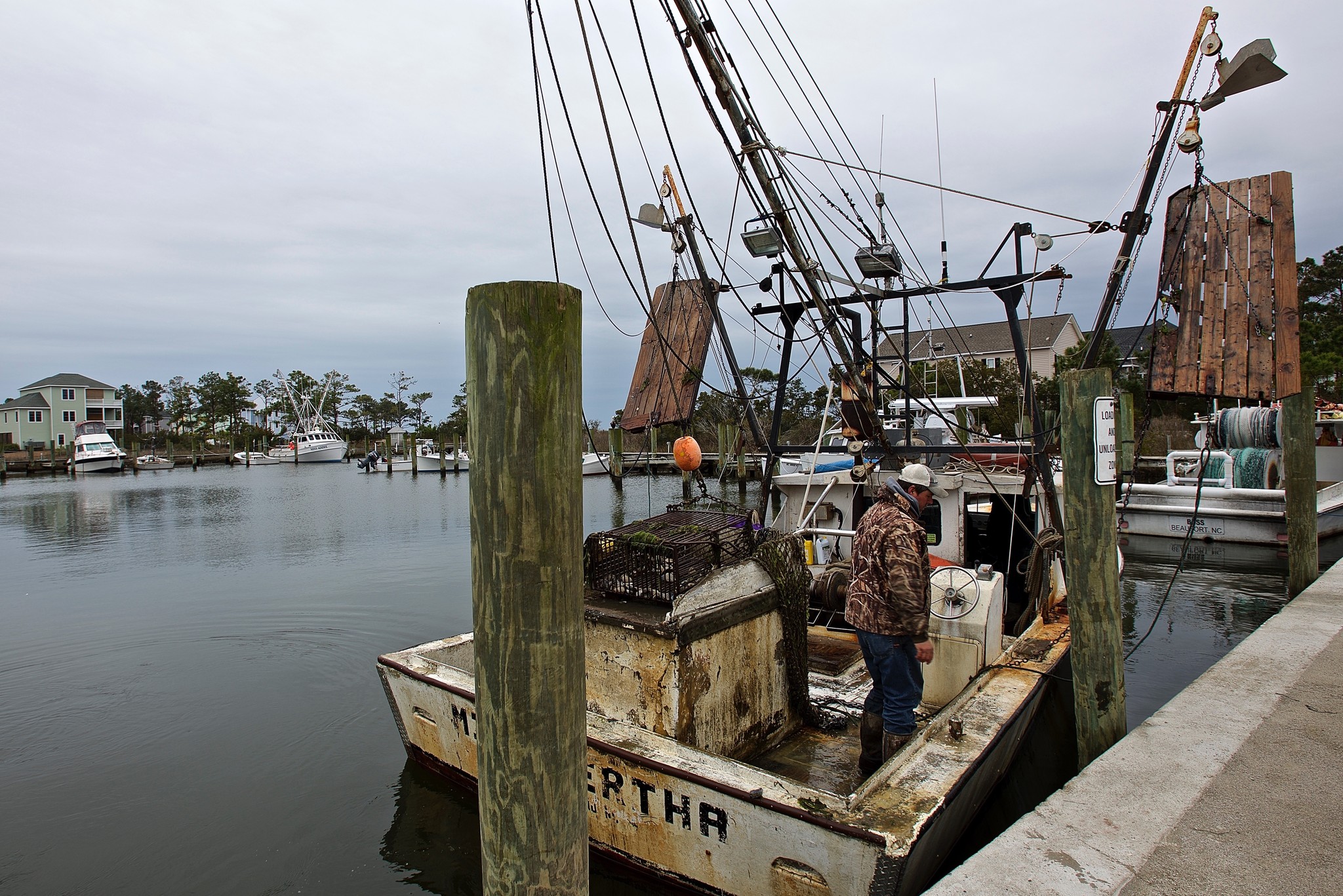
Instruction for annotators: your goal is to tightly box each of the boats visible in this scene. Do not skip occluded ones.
[1113,397,1343,542]
[582,451,610,474]
[268,368,349,461]
[380,2,1286,895]
[356,440,412,471]
[65,421,127,473]
[234,439,281,465]
[415,444,469,470]
[135,454,175,469]
[762,420,899,490]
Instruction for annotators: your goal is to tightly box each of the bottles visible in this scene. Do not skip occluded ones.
[804,539,813,565]
[816,537,832,565]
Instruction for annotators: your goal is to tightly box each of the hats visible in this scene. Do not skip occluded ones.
[898,463,949,498]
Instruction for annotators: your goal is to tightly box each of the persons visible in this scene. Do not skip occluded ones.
[853,464,936,779]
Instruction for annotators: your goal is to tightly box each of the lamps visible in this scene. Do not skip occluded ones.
[741,215,785,259]
[855,245,903,280]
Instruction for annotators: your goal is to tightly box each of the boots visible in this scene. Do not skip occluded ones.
[883,728,913,763]
[858,707,883,773]
